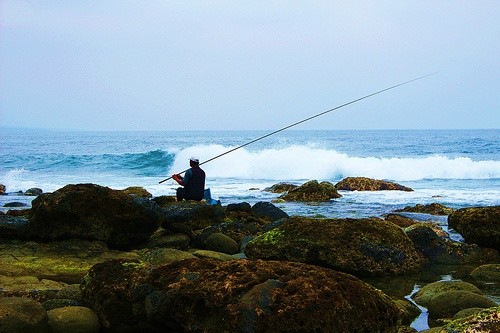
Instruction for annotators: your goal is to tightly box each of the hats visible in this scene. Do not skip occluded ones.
[188,155,199,163]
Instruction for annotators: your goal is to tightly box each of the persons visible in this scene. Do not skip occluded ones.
[173,157,206,203]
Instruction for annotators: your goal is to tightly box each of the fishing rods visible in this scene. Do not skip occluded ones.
[159,70,440,185]
